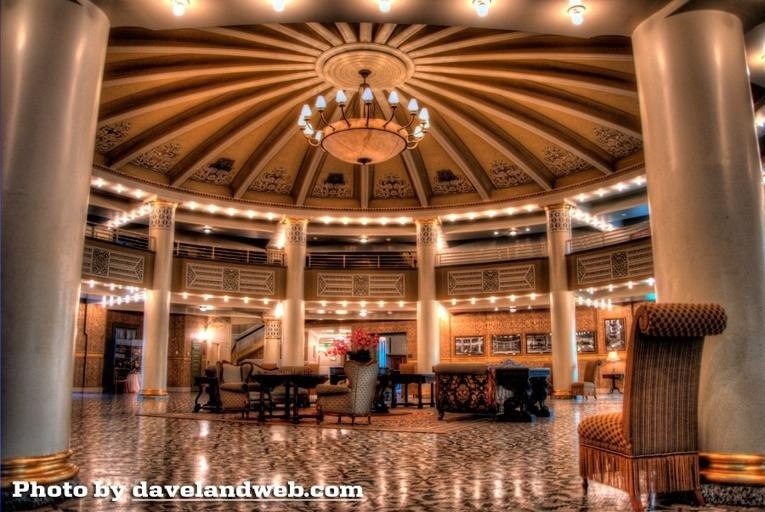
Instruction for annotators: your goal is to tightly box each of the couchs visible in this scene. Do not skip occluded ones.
[432,358,529,419]
[217,360,311,414]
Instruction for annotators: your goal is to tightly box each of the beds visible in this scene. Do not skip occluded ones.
[252,373,330,424]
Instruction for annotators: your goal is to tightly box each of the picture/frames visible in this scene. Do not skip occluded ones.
[453,334,487,357]
[575,328,599,355]
[525,332,552,354]
[604,316,627,352]
[490,332,523,356]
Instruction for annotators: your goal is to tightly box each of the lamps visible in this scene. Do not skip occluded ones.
[606,351,620,374]
[296,67,432,166]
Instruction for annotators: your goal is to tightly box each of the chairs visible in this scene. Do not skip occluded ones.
[578,304,729,512]
[572,359,603,401]
[315,358,379,425]
